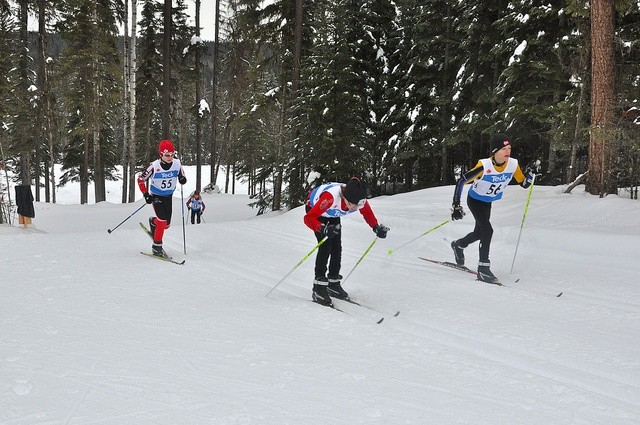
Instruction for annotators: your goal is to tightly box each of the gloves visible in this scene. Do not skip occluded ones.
[143,193,154,204]
[188,207,191,210]
[199,211,203,215]
[179,176,187,184]
[319,224,342,239]
[451,203,466,221]
[525,159,542,177]
[372,224,390,238]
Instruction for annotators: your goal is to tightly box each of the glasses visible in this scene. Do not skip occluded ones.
[164,152,175,157]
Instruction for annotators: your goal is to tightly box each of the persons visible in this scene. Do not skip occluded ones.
[303,177,387,306]
[186,190,205,224]
[138,139,187,257]
[451,134,541,283]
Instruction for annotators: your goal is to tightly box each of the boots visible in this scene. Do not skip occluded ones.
[152,241,168,259]
[327,274,348,300]
[312,277,331,304]
[477,259,498,284]
[451,238,468,265]
[149,216,157,238]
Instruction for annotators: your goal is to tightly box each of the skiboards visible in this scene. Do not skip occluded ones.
[139,222,185,265]
[311,296,400,324]
[418,256,562,297]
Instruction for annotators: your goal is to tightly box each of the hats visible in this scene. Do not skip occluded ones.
[159,139,175,158]
[490,132,512,155]
[194,190,199,195]
[342,177,367,205]
